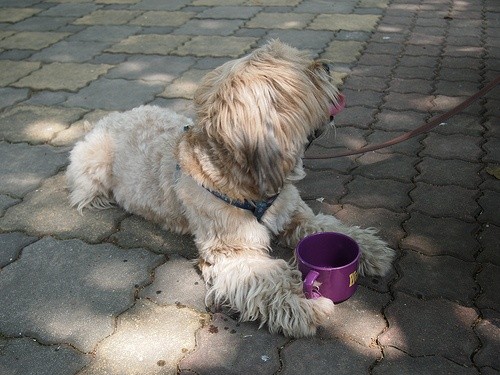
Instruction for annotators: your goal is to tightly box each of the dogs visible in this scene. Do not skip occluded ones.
[61,38,396,340]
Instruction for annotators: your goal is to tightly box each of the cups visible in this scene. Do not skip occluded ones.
[296,231,360,304]
